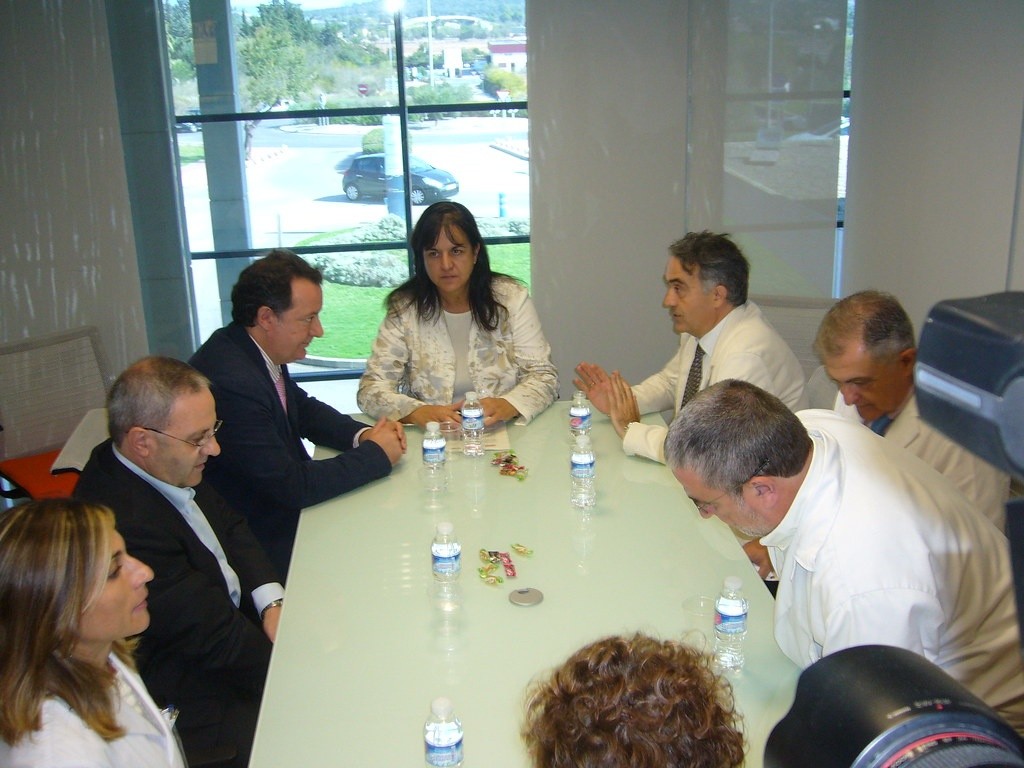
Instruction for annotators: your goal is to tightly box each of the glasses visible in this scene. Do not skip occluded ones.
[694,460,770,516]
[126,418,223,448]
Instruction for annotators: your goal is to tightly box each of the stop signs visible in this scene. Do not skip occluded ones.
[357,82,369,94]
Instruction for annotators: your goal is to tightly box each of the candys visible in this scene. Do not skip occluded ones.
[478,544,533,586]
[490,448,528,480]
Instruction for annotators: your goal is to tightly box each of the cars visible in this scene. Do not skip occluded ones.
[342,152,460,206]
[175,107,203,133]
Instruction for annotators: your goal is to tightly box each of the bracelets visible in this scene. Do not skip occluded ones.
[262,599,283,622]
[623,422,638,432]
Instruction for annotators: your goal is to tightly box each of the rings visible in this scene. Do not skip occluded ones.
[591,382,594,386]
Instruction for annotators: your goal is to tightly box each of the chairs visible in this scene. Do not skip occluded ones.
[0,325,113,500]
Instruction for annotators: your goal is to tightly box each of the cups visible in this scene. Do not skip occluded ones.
[440,421,461,462]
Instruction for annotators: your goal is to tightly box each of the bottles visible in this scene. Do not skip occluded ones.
[424,697,466,768]
[461,391,485,460]
[713,575,750,672]
[422,422,448,492]
[569,433,596,509]
[568,390,593,446]
[431,522,463,613]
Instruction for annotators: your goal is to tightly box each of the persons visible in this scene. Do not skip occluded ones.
[186,248,408,587]
[519,631,746,768]
[70,355,285,768]
[572,230,810,465]
[763,645,1024,768]
[0,498,189,768]
[663,378,1024,738]
[357,202,561,438]
[742,289,1012,599]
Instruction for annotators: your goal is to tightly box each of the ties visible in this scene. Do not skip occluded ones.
[871,415,895,438]
[274,372,289,419]
[680,342,706,411]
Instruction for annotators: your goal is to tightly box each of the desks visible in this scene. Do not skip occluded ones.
[245,397,806,768]
